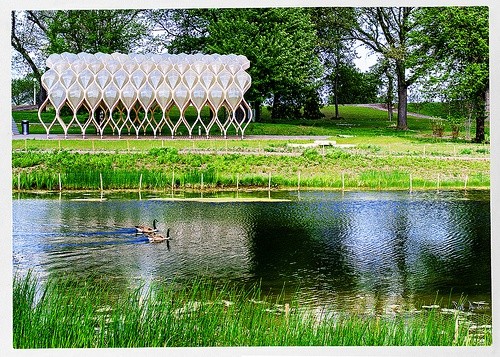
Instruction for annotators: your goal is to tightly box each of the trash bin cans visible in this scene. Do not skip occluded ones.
[21,119,29,135]
[246,100,256,123]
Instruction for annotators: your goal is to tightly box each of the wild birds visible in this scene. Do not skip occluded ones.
[145,229,173,243]
[135,219,159,233]
